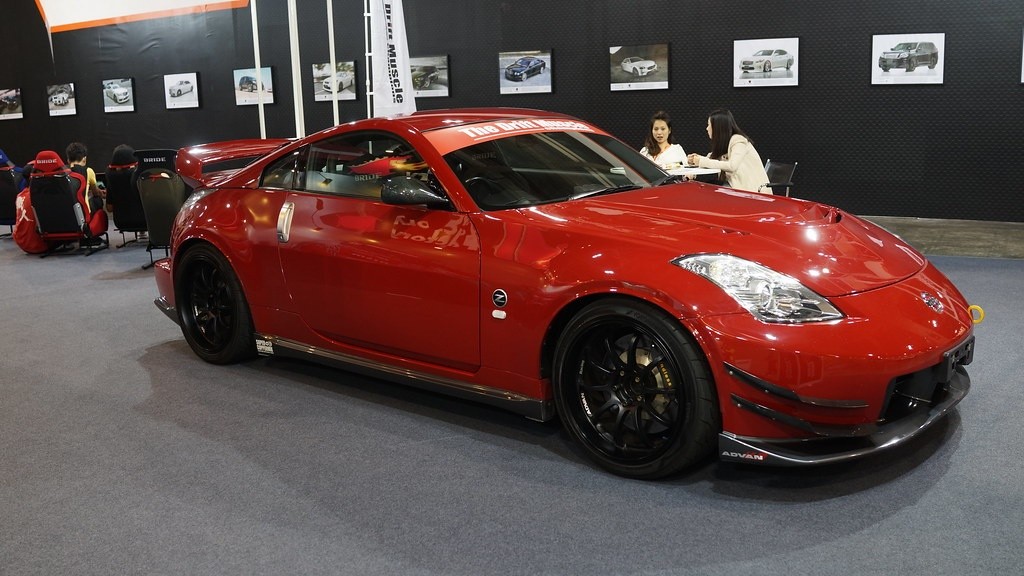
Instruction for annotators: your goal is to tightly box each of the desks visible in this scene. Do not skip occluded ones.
[666,164,721,185]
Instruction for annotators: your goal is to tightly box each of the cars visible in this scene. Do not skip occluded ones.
[106,84,129,104]
[740,49,794,73]
[169,80,193,97]
[505,57,546,82]
[152,106,984,480]
[323,70,353,93]
[411,65,439,90]
[622,57,657,77]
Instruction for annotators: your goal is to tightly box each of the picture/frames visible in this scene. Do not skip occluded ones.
[100,77,137,114]
[870,34,947,86]
[311,59,359,102]
[163,71,202,110]
[0,87,24,121]
[44,82,79,117]
[1017,26,1024,84]
[232,66,276,105]
[731,36,800,89]
[608,42,672,92]
[410,54,451,98]
[496,46,554,95]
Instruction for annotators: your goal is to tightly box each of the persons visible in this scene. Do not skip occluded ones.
[66,143,103,245]
[687,109,773,195]
[99,145,149,239]
[13,165,64,254]
[639,111,687,167]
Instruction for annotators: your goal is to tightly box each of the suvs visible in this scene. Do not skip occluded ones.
[879,42,938,72]
[240,77,264,92]
[52,90,69,105]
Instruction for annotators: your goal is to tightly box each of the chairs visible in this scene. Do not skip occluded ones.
[765,158,799,196]
[0,151,187,270]
